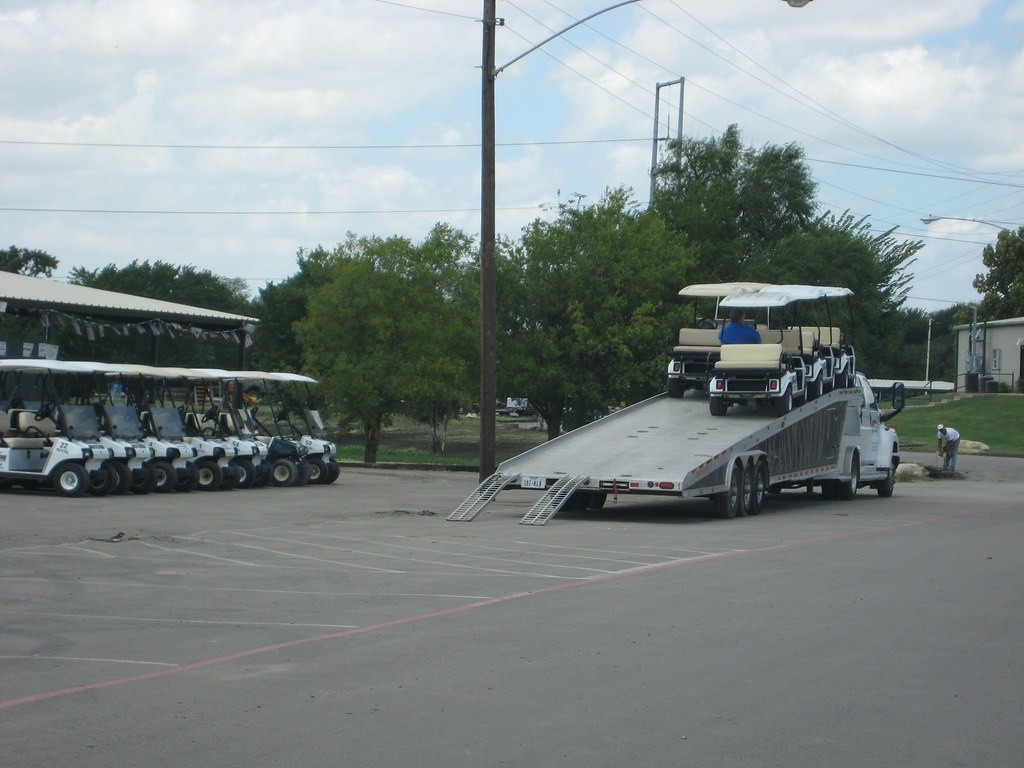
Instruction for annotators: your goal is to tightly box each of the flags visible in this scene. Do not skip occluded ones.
[149,325,160,336]
[38,313,50,327]
[71,321,82,335]
[123,325,128,335]
[245,333,253,348]
[135,324,145,333]
[97,326,104,337]
[86,326,94,340]
[231,332,240,344]
[220,332,229,339]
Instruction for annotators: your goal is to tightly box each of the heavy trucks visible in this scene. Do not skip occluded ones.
[445,373,905,526]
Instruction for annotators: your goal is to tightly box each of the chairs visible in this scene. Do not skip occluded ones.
[0,408,274,449]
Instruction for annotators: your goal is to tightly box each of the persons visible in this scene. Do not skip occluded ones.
[718,309,761,345]
[937,424,960,471]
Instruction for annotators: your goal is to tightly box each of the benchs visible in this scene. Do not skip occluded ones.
[673,322,842,374]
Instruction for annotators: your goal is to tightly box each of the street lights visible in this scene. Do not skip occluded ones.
[479,0,811,501]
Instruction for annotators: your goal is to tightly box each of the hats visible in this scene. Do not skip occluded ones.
[937,424,944,431]
